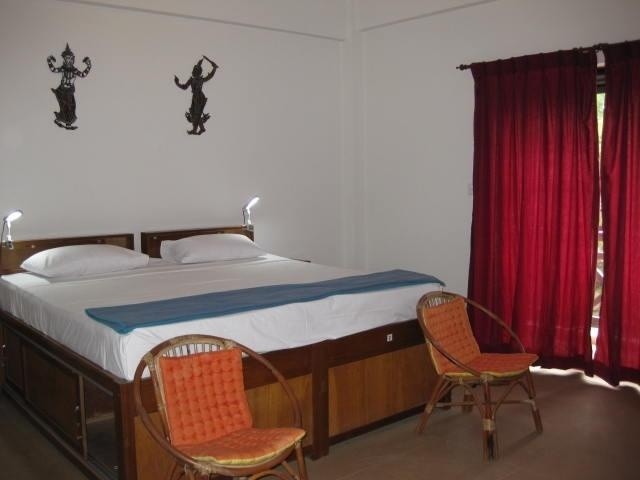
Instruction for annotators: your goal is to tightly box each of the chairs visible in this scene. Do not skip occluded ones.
[132,334,309,480]
[415,289,544,461]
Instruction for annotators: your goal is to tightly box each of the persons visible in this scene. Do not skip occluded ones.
[174,58,218,135]
[47,42,91,130]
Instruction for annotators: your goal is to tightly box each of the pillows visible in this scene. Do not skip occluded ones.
[158,233,268,265]
[19,244,154,278]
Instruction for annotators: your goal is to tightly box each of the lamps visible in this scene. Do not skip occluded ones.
[241,195,260,235]
[0,208,24,250]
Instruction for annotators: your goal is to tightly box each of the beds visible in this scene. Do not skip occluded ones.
[0,224,453,480]
